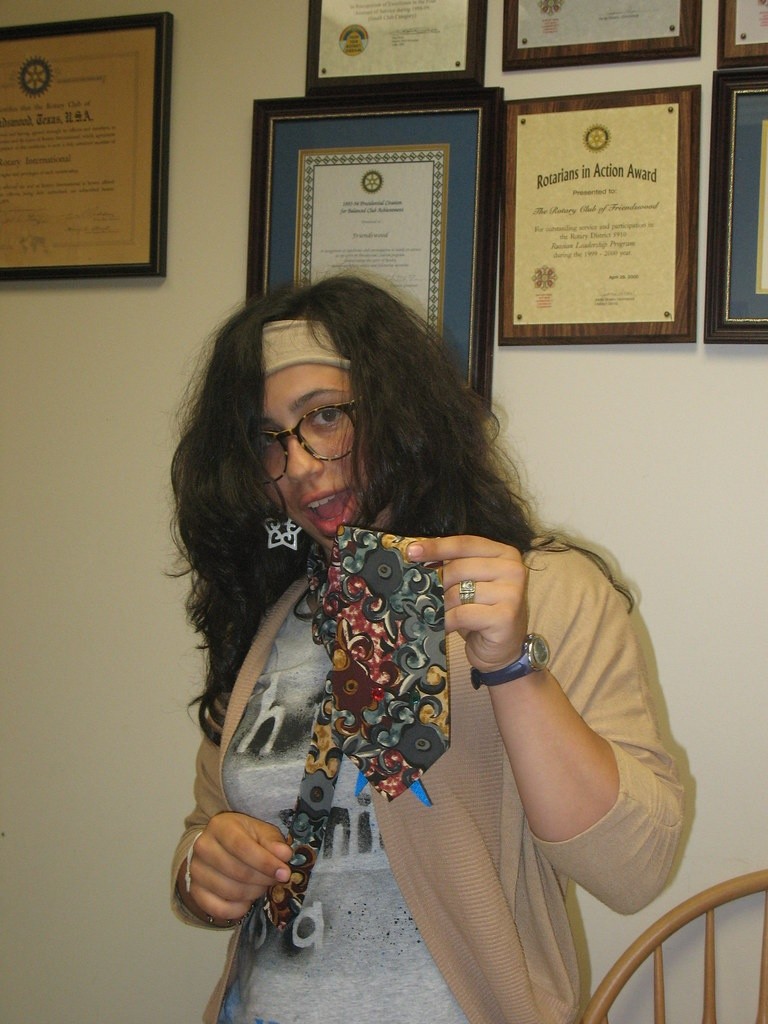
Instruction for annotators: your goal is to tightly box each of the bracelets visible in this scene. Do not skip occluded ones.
[185,831,255,923]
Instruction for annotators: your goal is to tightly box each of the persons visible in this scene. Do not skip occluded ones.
[168,277,696,1024]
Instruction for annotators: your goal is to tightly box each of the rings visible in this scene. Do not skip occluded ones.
[459,580,475,605]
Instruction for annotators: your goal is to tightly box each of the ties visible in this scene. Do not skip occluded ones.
[264,526,450,929]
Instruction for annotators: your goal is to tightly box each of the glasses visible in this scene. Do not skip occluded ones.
[254,393,362,486]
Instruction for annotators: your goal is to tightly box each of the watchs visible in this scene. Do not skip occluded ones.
[471,634,551,690]
